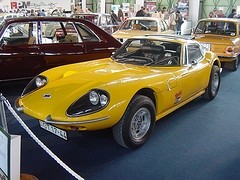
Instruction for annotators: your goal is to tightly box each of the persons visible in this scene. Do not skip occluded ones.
[0,3,240,35]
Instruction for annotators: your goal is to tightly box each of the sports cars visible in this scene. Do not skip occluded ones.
[14,35,221,150]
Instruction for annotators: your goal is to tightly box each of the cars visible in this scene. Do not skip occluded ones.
[0,17,128,103]
[189,18,240,70]
[112,17,174,44]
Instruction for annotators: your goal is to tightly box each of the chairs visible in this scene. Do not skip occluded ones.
[129,24,159,31]
[205,24,236,35]
[138,45,200,66]
[62,33,78,43]
